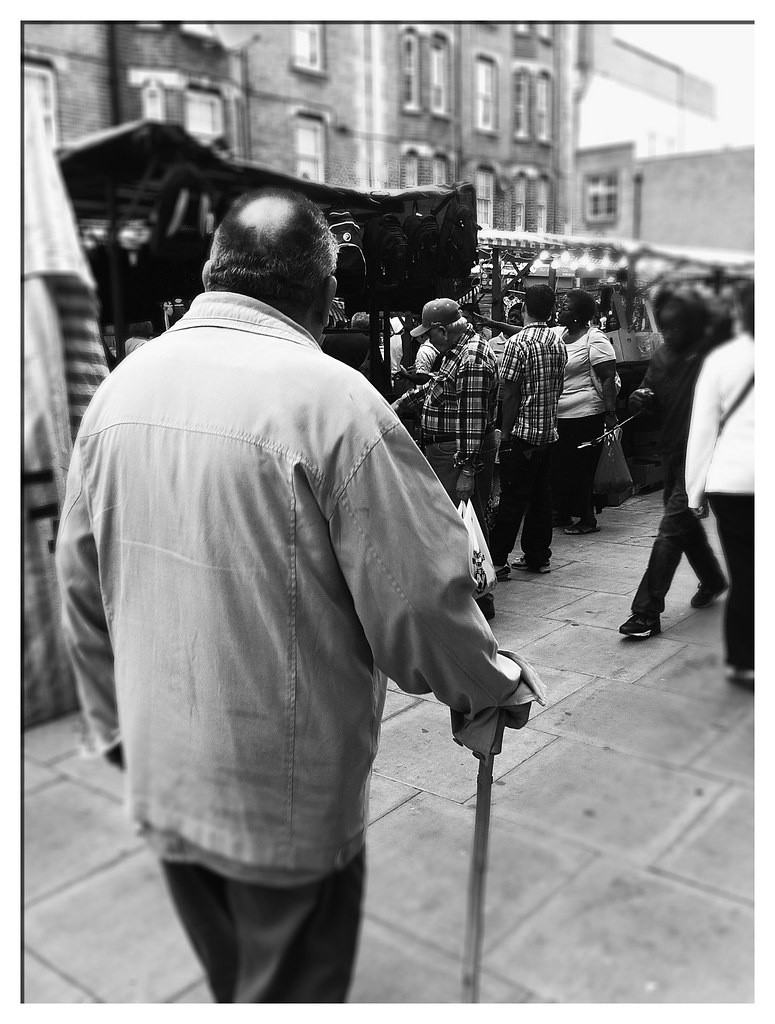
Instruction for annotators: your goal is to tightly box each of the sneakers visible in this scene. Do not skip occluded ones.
[511,554,552,574]
[691,575,730,609]
[723,666,755,690]
[619,612,662,639]
[494,561,512,582]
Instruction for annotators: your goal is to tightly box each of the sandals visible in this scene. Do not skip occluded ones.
[564,517,602,535]
[551,514,575,527]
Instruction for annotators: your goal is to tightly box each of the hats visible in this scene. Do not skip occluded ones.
[409,298,463,338]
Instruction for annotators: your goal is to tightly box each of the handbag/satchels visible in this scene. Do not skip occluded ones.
[457,498,498,601]
[590,424,634,507]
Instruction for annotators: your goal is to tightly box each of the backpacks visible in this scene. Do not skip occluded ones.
[144,170,220,295]
[401,196,440,290]
[322,198,368,301]
[435,193,484,281]
[360,203,419,306]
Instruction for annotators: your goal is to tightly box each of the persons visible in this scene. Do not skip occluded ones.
[52,184,549,1004]
[684,279,754,693]
[618,288,730,638]
[487,284,568,584]
[553,289,621,535]
